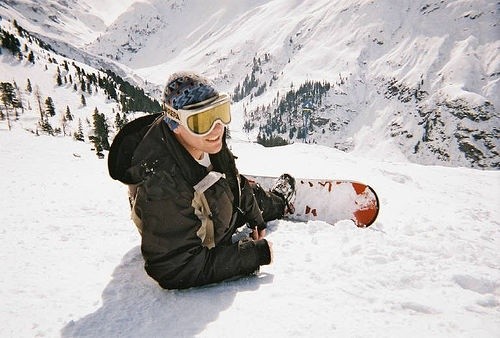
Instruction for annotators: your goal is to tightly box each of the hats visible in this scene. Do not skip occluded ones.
[162,71,220,131]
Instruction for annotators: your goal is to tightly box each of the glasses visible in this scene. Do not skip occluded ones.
[160,92,232,137]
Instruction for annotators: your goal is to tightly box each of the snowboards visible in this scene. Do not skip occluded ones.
[239,174,379,229]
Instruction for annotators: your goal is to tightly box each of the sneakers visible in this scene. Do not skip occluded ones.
[270,174,297,219]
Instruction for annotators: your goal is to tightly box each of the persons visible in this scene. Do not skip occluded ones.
[107,71,296,290]
